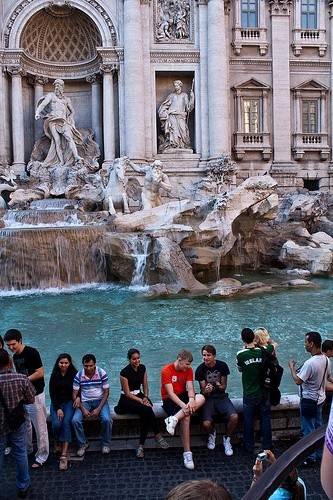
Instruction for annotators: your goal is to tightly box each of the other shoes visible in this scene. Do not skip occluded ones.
[263,443,275,451]
[243,447,253,453]
[77,442,90,456]
[54,440,61,451]
[4,446,12,455]
[102,445,110,454]
[298,459,316,468]
[32,461,41,468]
[18,484,32,498]
[59,456,67,471]
[316,457,321,464]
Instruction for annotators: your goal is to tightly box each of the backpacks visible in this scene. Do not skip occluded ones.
[257,345,284,390]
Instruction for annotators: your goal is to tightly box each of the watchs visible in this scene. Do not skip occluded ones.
[253,478,258,482]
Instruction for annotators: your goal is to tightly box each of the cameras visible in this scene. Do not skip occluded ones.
[258,452,267,461]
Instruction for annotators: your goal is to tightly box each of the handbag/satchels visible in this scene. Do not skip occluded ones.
[301,398,317,418]
[6,399,26,431]
[270,388,281,406]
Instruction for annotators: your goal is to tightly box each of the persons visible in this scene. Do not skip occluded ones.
[35,79,83,166]
[129,159,171,209]
[160,349,205,469]
[49,353,81,470]
[0,328,49,498]
[71,354,111,456]
[195,345,238,456]
[236,327,278,469]
[158,5,190,40]
[321,399,333,500]
[251,450,307,500]
[158,80,194,148]
[165,478,234,500]
[114,348,169,458]
[289,331,333,466]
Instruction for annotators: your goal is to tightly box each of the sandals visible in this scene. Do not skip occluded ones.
[136,447,144,458]
[155,437,169,449]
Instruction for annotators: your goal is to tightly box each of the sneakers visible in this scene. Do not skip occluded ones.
[183,451,195,469]
[223,435,234,456]
[207,428,216,449]
[164,415,178,436]
